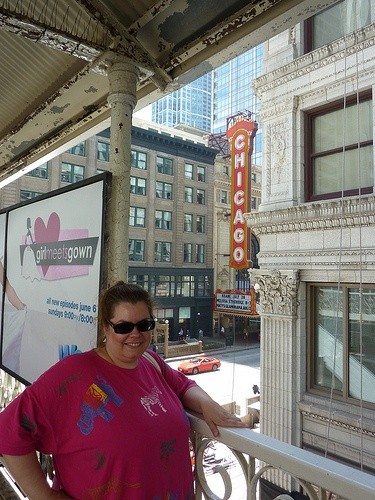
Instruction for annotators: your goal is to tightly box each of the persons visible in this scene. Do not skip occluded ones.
[0,283,248,500]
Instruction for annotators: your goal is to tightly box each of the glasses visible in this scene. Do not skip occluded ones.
[104,311,156,335]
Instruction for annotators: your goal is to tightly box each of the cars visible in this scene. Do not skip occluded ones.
[177,356,222,375]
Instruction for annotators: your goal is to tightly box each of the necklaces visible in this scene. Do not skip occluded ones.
[104,345,118,367]
